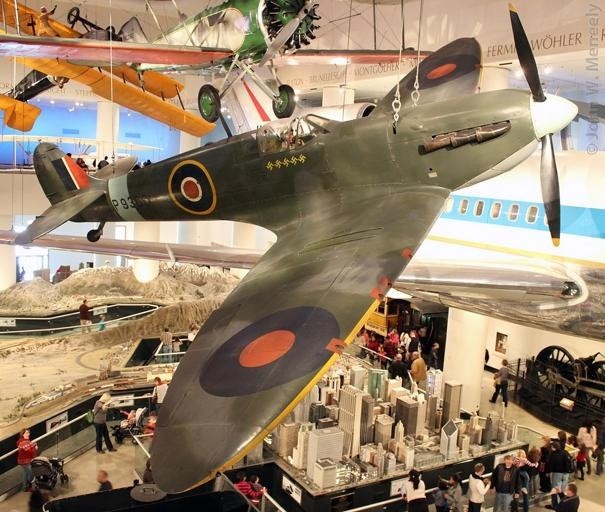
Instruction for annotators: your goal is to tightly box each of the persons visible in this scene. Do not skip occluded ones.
[96,314,111,331]
[78,298,98,331]
[144,458,154,482]
[157,324,198,363]
[109,410,138,431]
[234,474,266,498]
[17,429,40,492]
[26,4,61,38]
[97,470,113,489]
[389,421,603,511]
[276,127,304,151]
[90,389,118,455]
[148,377,168,413]
[488,360,510,409]
[59,153,153,173]
[354,326,441,386]
[250,476,264,502]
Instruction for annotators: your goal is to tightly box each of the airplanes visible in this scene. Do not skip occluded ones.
[1,0,442,123]
[12,4,582,494]
[2,3,223,137]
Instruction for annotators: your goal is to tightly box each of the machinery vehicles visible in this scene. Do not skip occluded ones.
[532,343,605,414]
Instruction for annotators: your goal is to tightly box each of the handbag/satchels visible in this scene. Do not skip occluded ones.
[151,395,157,404]
[86,409,94,423]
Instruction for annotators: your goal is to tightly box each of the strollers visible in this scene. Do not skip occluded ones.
[110,407,148,446]
[30,454,69,489]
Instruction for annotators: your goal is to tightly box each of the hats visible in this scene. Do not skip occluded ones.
[98,392,112,403]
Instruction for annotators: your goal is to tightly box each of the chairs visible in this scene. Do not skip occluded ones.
[263,136,282,154]
